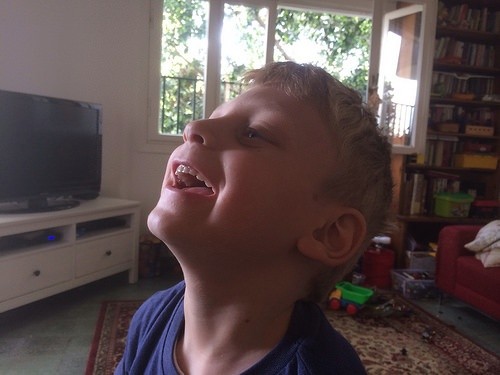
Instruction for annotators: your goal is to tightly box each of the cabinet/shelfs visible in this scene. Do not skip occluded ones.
[387,0,500,268]
[0,197,140,314]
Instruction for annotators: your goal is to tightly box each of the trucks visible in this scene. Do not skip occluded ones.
[329,281,374,315]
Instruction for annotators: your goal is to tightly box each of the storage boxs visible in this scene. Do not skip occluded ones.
[390,251,437,298]
[433,191,500,218]
[453,151,500,169]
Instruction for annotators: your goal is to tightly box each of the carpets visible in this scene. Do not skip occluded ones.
[84,294,500,375]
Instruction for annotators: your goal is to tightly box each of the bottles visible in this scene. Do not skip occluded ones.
[403,172,427,217]
[361,236,396,291]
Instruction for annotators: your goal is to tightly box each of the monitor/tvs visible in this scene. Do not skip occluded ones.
[0,89,102,212]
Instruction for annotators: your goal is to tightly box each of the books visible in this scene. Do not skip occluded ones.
[430,0,500,100]
[424,134,458,167]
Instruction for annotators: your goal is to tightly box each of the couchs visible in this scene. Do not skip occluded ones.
[434,224,500,320]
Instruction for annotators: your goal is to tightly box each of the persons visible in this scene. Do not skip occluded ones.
[113,60,394,375]
